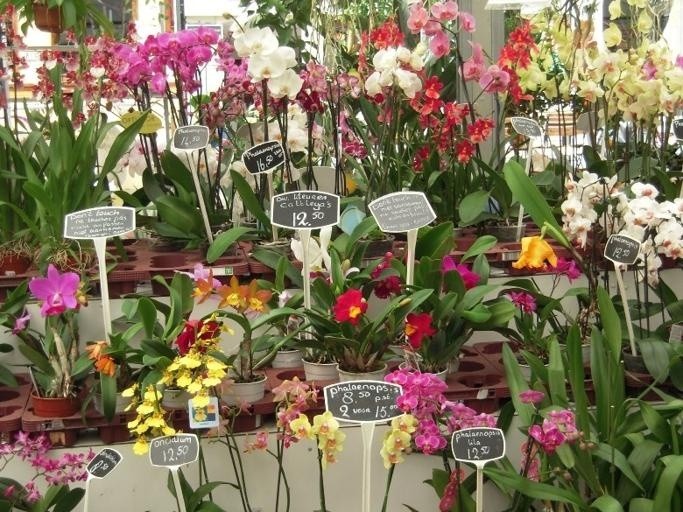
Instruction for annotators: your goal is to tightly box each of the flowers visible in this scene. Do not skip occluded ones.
[0,1,683,512]
